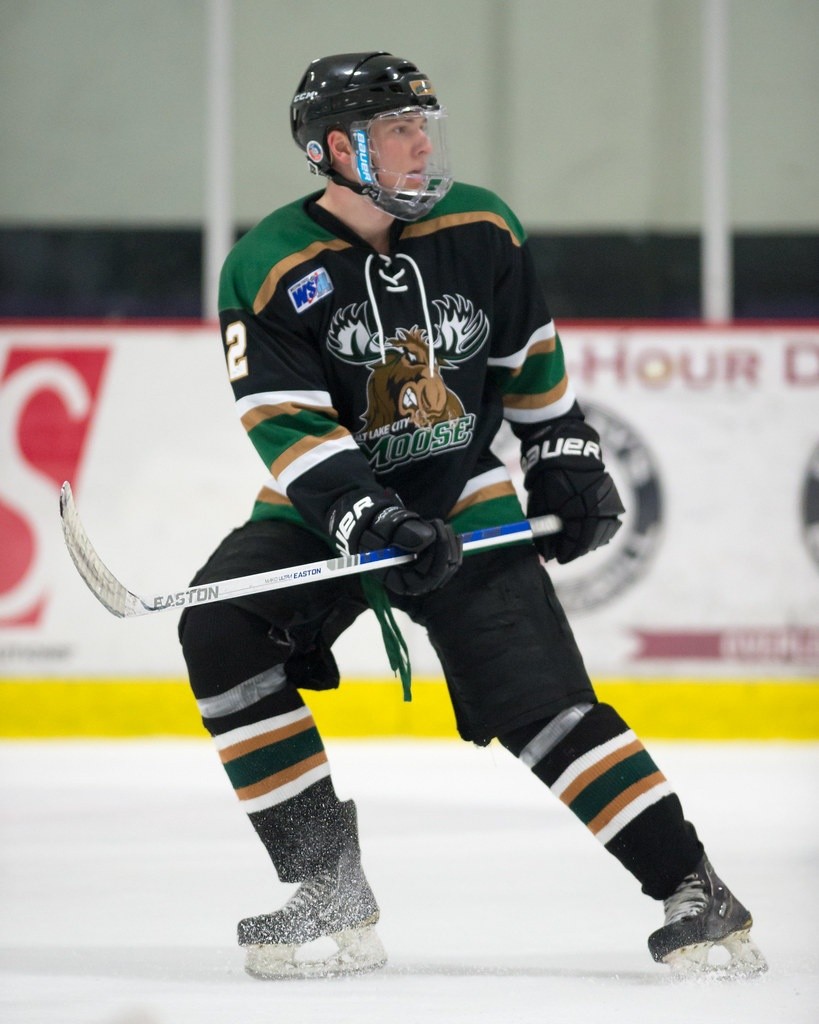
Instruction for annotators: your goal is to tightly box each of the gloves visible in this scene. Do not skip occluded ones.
[321,482,463,595]
[520,420,625,564]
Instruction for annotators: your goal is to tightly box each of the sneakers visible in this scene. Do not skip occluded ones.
[648,852,768,981]
[238,799,388,981]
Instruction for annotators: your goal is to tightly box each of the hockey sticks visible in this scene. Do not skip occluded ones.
[57,476,564,623]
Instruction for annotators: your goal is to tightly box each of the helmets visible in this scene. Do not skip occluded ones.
[290,51,453,221]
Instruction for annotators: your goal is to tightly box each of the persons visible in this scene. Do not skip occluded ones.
[178,50,768,981]
